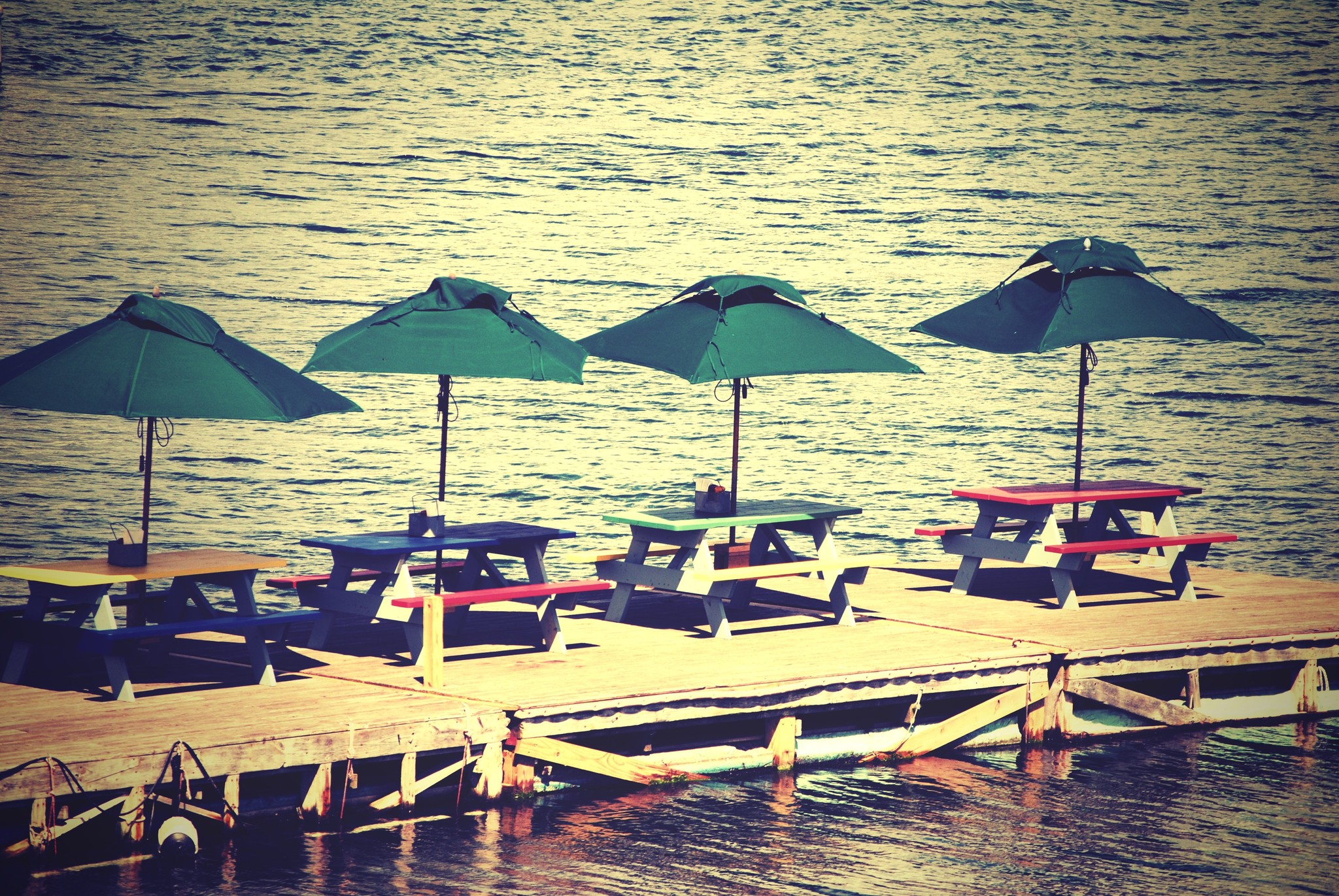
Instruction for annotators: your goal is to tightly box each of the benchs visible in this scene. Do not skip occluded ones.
[1042,532,1238,553]
[391,580,610,607]
[694,553,898,581]
[94,609,321,641]
[0,589,173,615]
[567,538,753,563]
[266,561,465,591]
[910,517,1089,536]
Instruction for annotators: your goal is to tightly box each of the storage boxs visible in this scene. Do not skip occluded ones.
[407,494,446,538]
[106,521,147,566]
[693,477,731,514]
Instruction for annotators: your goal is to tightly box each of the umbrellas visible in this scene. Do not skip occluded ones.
[299,273,590,597]
[909,237,1266,544]
[0,285,364,566]
[574,268,926,543]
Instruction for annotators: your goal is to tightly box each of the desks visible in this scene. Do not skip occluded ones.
[299,518,577,667]
[0,548,287,702]
[602,497,861,642]
[949,479,1202,610]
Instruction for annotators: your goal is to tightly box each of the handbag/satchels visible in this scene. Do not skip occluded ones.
[408,494,446,537]
[107,522,145,566]
[693,478,731,513]
